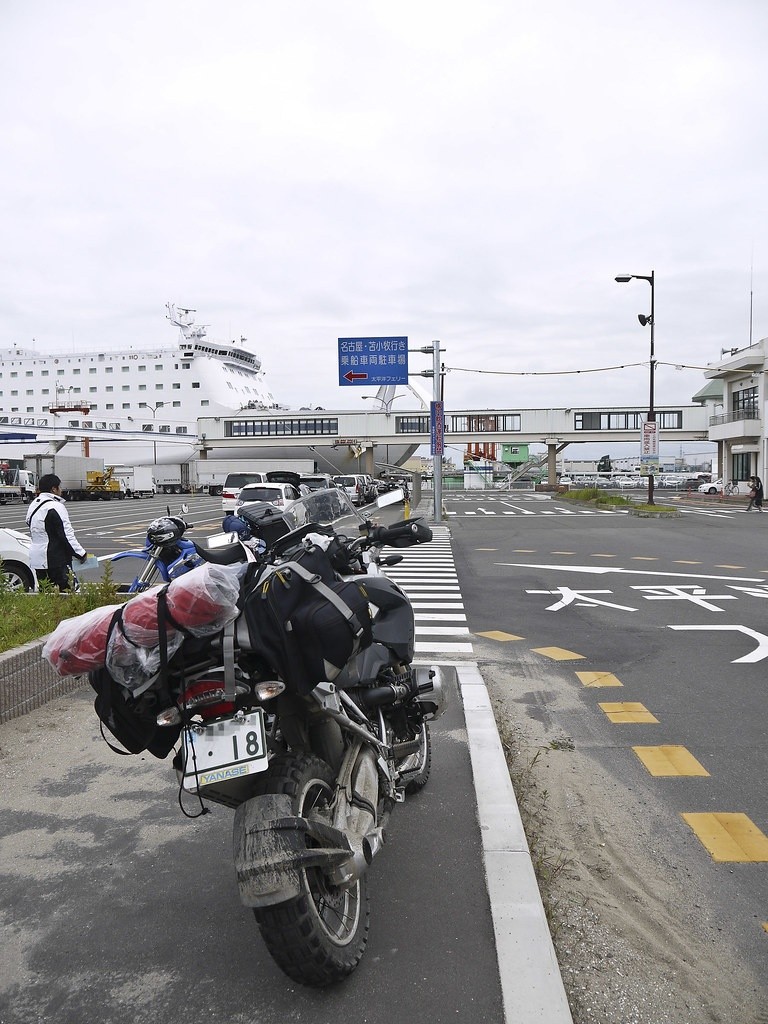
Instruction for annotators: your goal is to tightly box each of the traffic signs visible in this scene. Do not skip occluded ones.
[339,337,409,387]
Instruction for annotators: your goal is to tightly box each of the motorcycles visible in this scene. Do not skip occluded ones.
[112,487,448,989]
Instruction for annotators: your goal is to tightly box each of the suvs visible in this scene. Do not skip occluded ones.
[300,473,342,520]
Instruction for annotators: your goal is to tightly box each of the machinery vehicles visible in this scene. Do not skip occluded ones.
[87,466,127,501]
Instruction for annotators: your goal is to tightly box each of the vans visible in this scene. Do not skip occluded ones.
[233,482,309,530]
[332,476,366,507]
[222,471,267,517]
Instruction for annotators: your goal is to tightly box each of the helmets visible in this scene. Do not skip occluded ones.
[147,518,187,547]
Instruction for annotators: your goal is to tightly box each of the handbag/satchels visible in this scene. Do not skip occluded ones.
[88,639,209,760]
[222,515,251,540]
[239,543,372,698]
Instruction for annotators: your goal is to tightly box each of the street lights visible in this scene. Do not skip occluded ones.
[613,269,656,507]
[361,395,408,465]
[143,401,172,465]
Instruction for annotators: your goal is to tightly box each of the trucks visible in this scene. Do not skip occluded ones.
[0,468,40,505]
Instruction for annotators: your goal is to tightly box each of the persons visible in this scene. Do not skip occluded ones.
[25,473,87,593]
[746,476,764,513]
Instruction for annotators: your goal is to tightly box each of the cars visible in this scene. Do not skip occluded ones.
[558,475,688,488]
[697,478,723,495]
[265,470,311,497]
[357,475,399,505]
[0,526,40,593]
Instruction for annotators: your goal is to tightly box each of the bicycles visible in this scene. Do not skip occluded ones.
[724,479,739,497]
